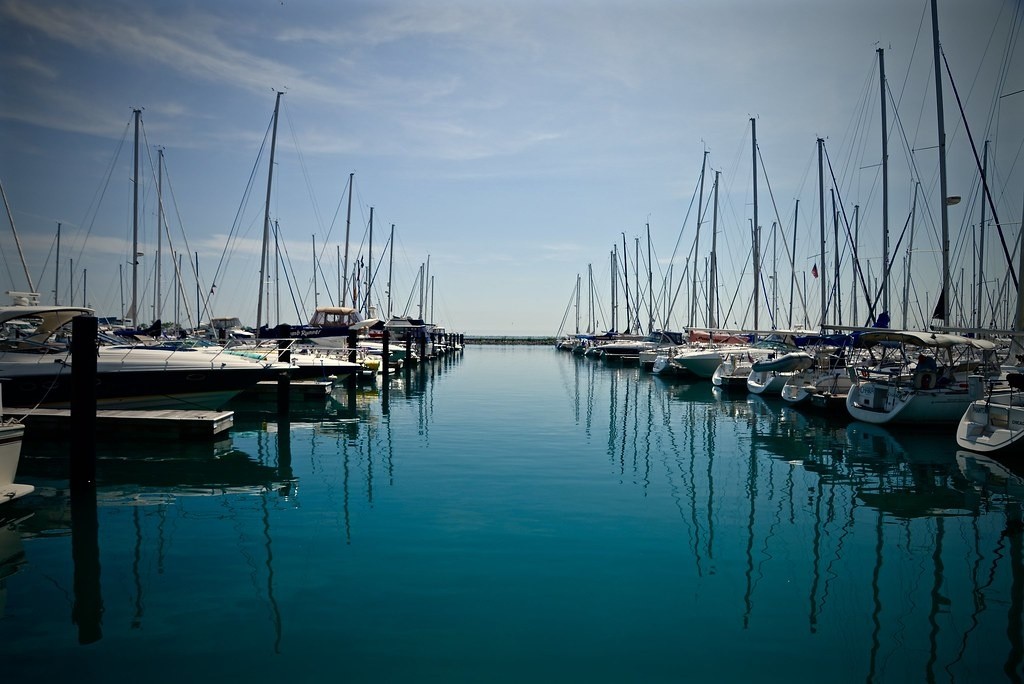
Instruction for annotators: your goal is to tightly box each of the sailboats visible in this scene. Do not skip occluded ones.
[554,1,1023,456]
[0,81,467,517]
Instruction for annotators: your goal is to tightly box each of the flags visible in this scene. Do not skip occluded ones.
[811,257,819,278]
[931,287,945,320]
